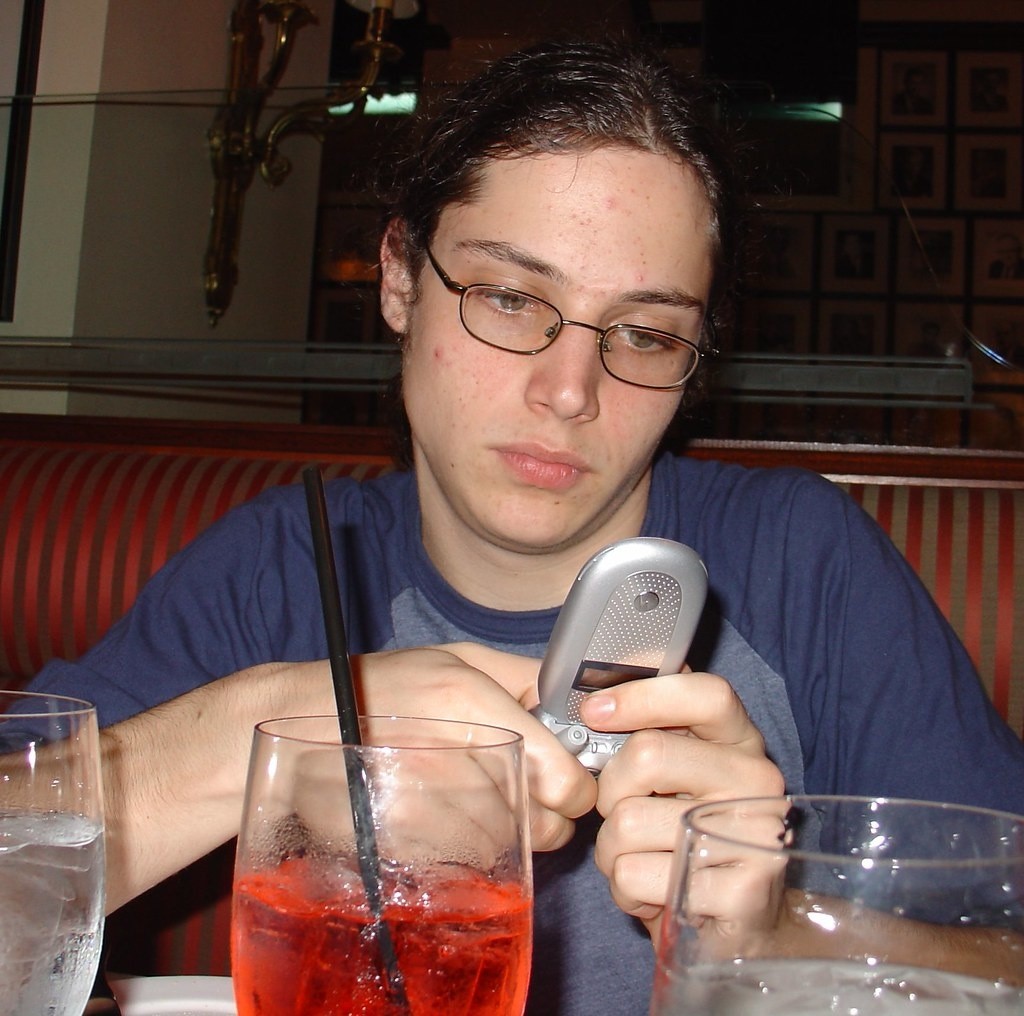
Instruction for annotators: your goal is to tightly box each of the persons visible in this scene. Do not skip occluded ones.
[0,37,1024,1016]
[754,63,1024,452]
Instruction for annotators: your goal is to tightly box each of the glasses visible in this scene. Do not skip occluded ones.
[420,227,719,389]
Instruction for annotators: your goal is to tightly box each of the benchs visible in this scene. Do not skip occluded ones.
[0,410,1024,980]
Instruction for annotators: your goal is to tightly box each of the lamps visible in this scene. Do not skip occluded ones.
[201,0,418,326]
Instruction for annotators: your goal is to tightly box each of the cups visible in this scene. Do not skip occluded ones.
[649,796,1024,1016]
[233,717,534,1016]
[0,692,107,1015]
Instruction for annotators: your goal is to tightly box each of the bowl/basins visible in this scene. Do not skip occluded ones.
[109,976,236,1016]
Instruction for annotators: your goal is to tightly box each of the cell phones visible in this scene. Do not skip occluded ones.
[519,537,710,780]
